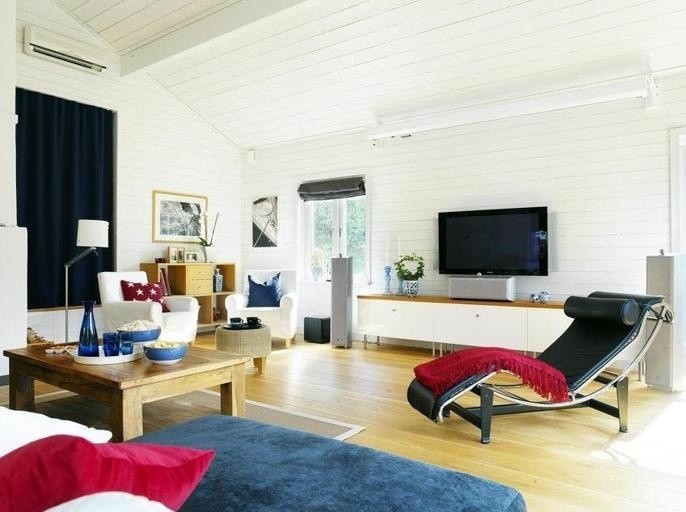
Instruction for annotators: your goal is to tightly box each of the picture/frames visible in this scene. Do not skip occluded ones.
[152,190,208,242]
[168,247,184,263]
[184,252,199,263]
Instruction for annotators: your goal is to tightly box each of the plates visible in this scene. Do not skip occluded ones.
[221,324,264,330]
[66,343,146,366]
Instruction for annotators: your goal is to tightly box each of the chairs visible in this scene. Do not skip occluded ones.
[405,291,672,444]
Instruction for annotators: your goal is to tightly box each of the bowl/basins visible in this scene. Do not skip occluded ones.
[143,345,187,365]
[116,328,161,342]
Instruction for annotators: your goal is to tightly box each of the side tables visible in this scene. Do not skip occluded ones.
[215,325,271,374]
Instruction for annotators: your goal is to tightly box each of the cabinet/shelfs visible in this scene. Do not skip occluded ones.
[140,263,237,328]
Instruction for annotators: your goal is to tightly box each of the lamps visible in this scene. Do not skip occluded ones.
[59,219,109,344]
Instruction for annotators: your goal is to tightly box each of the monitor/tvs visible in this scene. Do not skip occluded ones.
[438,206,549,277]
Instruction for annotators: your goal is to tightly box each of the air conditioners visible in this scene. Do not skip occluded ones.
[22,23,108,75]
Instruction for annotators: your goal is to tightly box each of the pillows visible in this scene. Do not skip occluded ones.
[121,280,171,312]
[247,272,281,307]
[0,435,213,511]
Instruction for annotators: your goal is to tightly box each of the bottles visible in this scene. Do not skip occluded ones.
[78,301,99,357]
[213,269,223,292]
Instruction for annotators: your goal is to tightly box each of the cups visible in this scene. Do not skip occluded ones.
[103,333,119,356]
[231,324,243,329]
[120,334,134,355]
[230,317,242,322]
[247,316,261,328]
[155,257,166,265]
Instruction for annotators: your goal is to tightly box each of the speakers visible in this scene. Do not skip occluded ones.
[645,255,685,393]
[330,257,353,349]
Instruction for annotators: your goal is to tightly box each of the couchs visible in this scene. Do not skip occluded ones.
[97,271,198,345]
[0,406,527,509]
[224,269,296,348]
